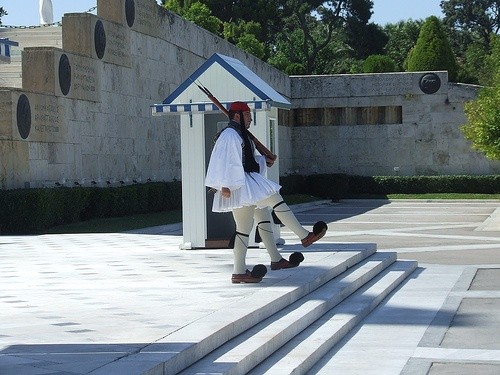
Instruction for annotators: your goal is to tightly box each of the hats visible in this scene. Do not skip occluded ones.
[229,101,250,111]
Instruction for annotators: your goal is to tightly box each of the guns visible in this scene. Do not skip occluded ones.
[188,75,278,167]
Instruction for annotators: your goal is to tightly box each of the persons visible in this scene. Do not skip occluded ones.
[252,138,328,248]
[204,102,305,283]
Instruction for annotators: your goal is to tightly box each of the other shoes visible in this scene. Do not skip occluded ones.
[232,264,267,283]
[301,221,327,248]
[271,252,304,270]
